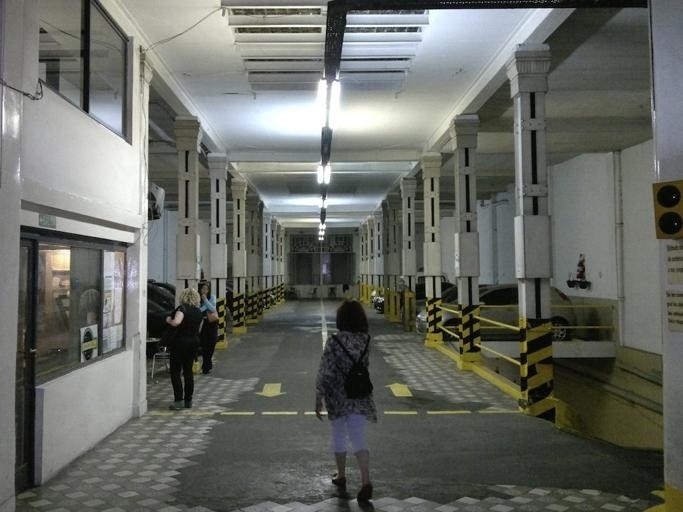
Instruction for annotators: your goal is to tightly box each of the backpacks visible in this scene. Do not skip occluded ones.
[331,334,373,401]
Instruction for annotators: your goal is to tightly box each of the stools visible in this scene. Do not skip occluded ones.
[151,352,170,379]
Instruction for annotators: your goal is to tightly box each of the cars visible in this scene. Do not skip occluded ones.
[373,280,456,313]
[148,279,232,357]
[416,282,576,340]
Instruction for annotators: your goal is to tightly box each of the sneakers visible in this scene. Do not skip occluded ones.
[201,364,213,374]
[356,483,373,504]
[169,399,193,409]
[332,473,347,486]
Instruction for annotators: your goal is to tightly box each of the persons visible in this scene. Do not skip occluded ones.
[162,288,203,411]
[315,298,378,502]
[196,282,218,372]
